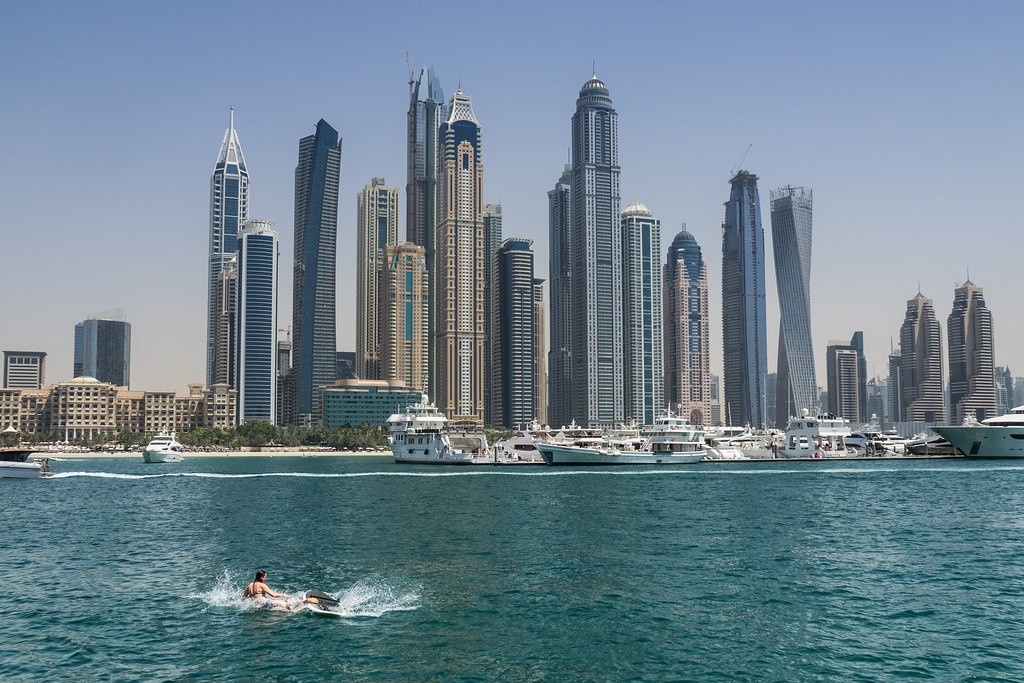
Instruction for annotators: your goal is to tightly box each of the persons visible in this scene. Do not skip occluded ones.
[817,437,885,458]
[41,458,51,477]
[242,570,319,604]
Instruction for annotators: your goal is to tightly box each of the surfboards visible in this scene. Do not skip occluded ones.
[302,589,346,616]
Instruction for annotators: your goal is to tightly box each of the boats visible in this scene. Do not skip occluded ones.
[383,392,923,464]
[143,435,185,463]
[0,458,50,479]
[926,405,1024,459]
